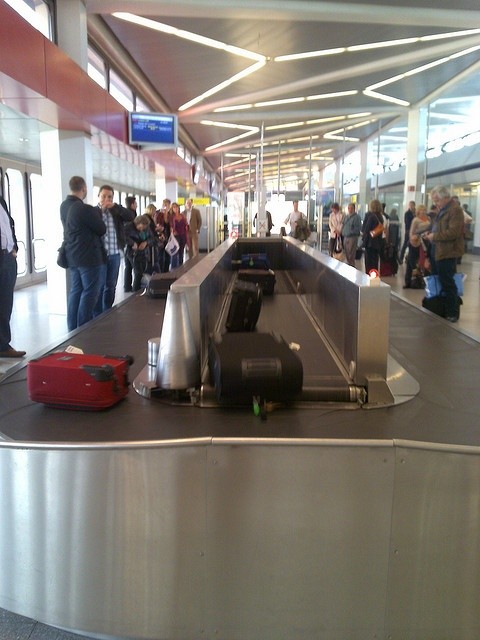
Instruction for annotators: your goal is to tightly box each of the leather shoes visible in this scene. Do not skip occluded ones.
[1,347,27,358]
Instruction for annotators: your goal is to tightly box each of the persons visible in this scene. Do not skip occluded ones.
[462,204,473,253]
[142,204,164,272]
[361,199,384,276]
[386,209,398,251]
[94,185,131,315]
[124,214,155,270]
[60,176,106,332]
[341,203,361,266]
[170,203,187,269]
[450,196,472,225]
[327,203,345,258]
[374,203,388,263]
[427,204,438,221]
[1,197,27,358]
[123,197,145,292]
[398,201,416,265]
[405,205,431,288]
[284,201,307,239]
[181,199,202,263]
[422,185,464,322]
[155,199,172,271]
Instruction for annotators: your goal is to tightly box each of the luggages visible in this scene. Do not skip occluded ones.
[207,332,304,403]
[237,269,275,295]
[380,238,394,275]
[143,273,179,298]
[27,351,135,412]
[225,278,263,330]
[241,253,271,269]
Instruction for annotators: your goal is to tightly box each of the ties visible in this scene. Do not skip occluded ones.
[0,196,18,253]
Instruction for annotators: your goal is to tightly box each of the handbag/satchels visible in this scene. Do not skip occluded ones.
[367,213,385,238]
[57,243,68,268]
[423,272,466,298]
[126,246,148,263]
[422,290,463,319]
[410,269,426,289]
